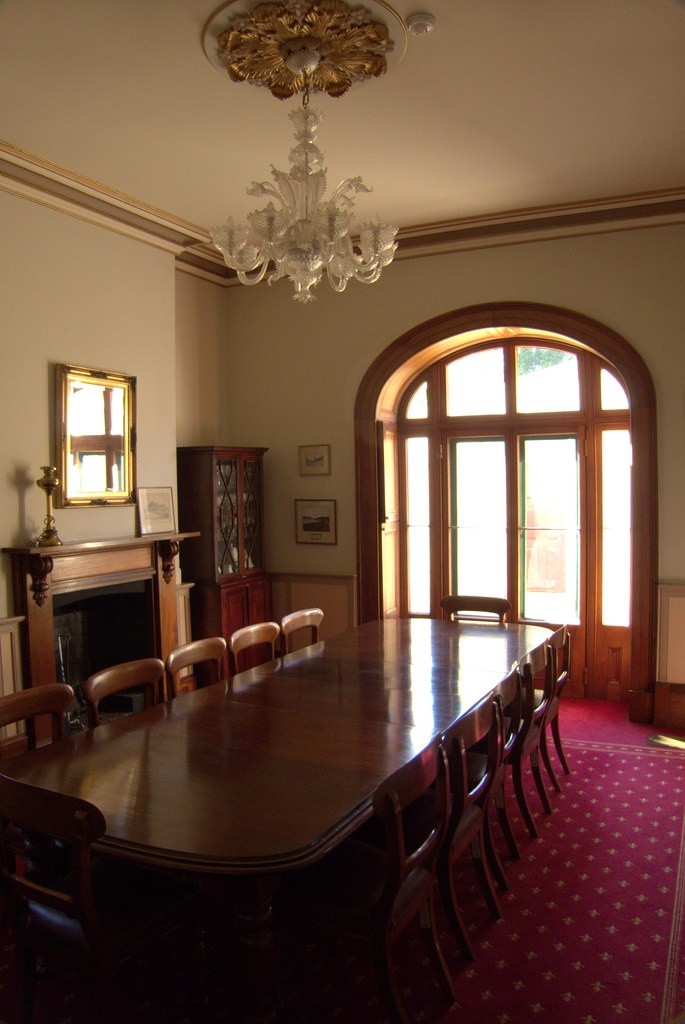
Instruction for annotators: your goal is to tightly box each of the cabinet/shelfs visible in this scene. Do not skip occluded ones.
[178,445,273,684]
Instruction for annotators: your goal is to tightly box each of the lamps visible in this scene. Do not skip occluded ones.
[201,0,409,301]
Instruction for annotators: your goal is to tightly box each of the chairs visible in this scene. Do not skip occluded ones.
[0,597,567,1024]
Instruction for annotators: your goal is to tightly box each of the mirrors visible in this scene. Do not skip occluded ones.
[47,360,137,506]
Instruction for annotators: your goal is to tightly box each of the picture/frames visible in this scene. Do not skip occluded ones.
[294,498,337,545]
[134,486,175,536]
[297,443,332,477]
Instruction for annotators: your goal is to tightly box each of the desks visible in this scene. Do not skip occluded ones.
[1,615,572,951]
